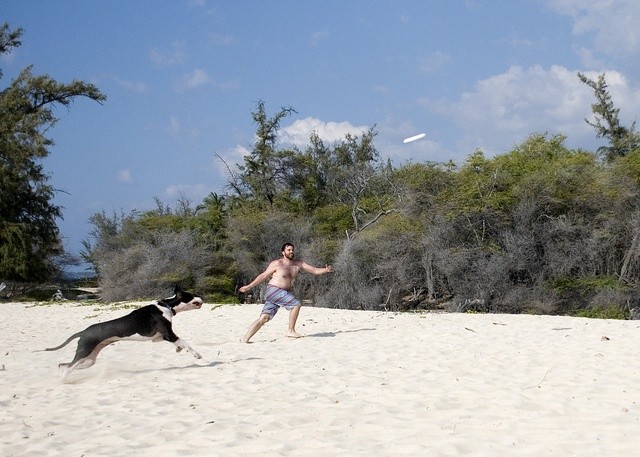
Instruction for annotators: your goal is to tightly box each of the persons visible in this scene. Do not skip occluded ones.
[239,242,333,343]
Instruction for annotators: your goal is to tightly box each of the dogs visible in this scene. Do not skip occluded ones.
[44,285,204,383]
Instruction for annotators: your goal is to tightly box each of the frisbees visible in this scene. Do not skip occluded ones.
[403,133,426,144]
